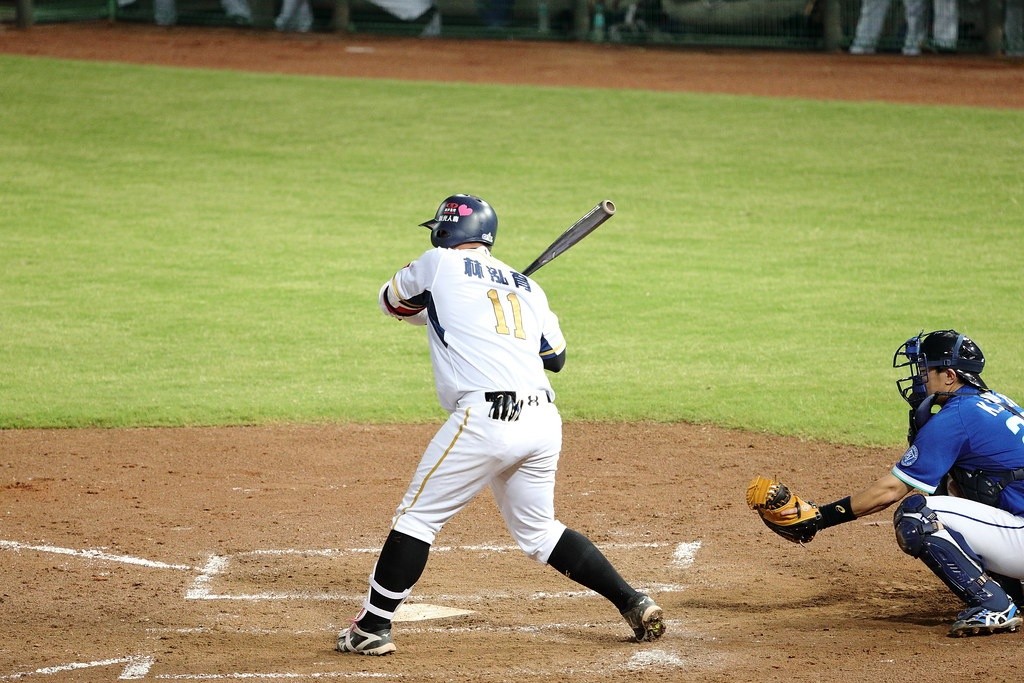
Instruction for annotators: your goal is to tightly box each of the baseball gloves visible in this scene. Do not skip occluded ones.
[745,474,818,547]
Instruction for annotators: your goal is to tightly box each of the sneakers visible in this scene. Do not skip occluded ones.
[621,593,666,642]
[952,596,1024,637]
[337,613,396,656]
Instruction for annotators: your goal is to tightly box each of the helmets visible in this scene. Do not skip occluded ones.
[920,330,988,391]
[418,194,497,248]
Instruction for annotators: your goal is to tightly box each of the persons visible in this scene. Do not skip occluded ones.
[334,196,667,656]
[804,0,957,56]
[746,329,1024,637]
[118,0,515,38]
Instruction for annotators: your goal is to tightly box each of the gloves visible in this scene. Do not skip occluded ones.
[488,394,521,420]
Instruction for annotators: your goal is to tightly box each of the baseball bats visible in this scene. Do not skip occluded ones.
[521,199,616,279]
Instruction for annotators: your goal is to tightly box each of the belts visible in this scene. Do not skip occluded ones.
[485,392,552,404]
